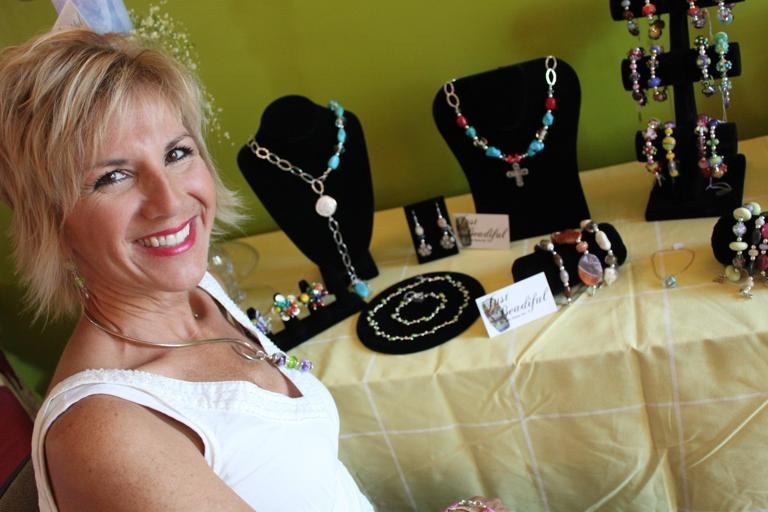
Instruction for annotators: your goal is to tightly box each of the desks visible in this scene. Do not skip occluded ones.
[204,135,768,512]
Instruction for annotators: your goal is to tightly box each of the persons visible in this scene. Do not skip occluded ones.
[1,25,509,512]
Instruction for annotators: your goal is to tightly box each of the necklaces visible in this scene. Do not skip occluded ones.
[79,291,318,371]
[247,101,369,298]
[368,273,471,341]
[443,54,559,188]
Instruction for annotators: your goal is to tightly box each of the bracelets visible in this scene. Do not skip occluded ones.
[578,220,620,287]
[694,115,713,181]
[641,1,665,40]
[552,230,604,297]
[758,223,768,279]
[652,242,696,288]
[708,117,729,182]
[713,200,761,284]
[688,1,709,29]
[391,290,447,325]
[736,211,767,297]
[622,1,640,36]
[713,31,735,108]
[659,122,686,177]
[540,238,576,306]
[643,118,664,181]
[649,41,669,104]
[444,499,498,512]
[718,2,735,25]
[628,46,650,105]
[694,35,717,98]
